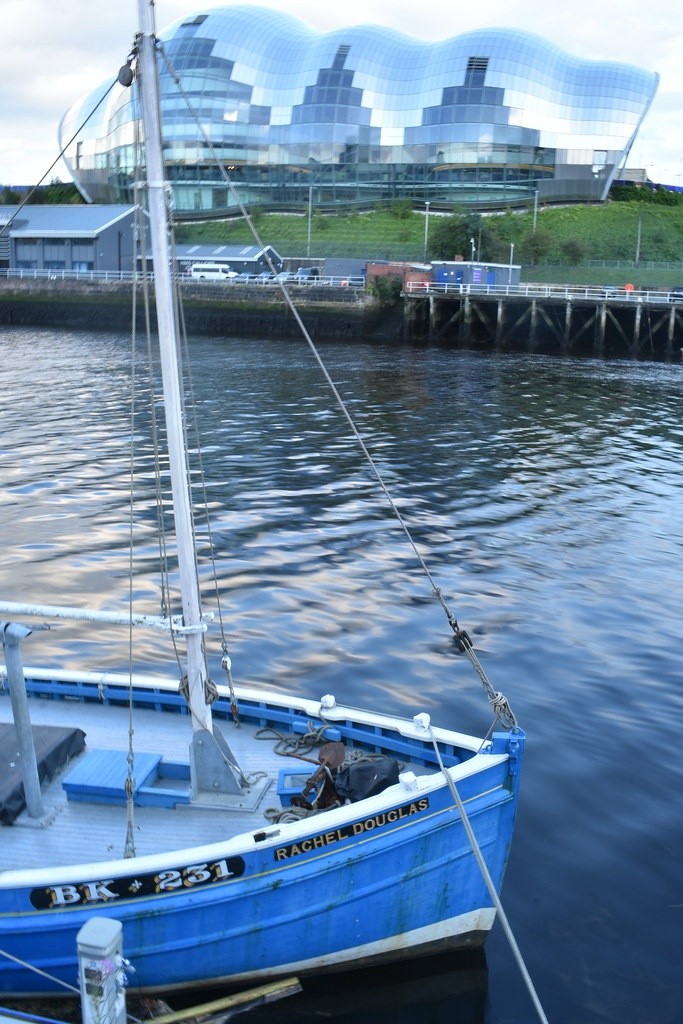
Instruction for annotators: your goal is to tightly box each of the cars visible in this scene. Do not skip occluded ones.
[235,273,257,283]
[274,271,296,285]
[668,286,683,302]
[602,285,618,298]
[254,271,276,285]
[347,274,366,287]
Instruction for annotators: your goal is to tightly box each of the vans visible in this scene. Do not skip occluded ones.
[192,263,238,280]
[294,268,319,283]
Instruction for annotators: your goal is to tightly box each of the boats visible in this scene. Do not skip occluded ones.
[0,0,558,1024]
